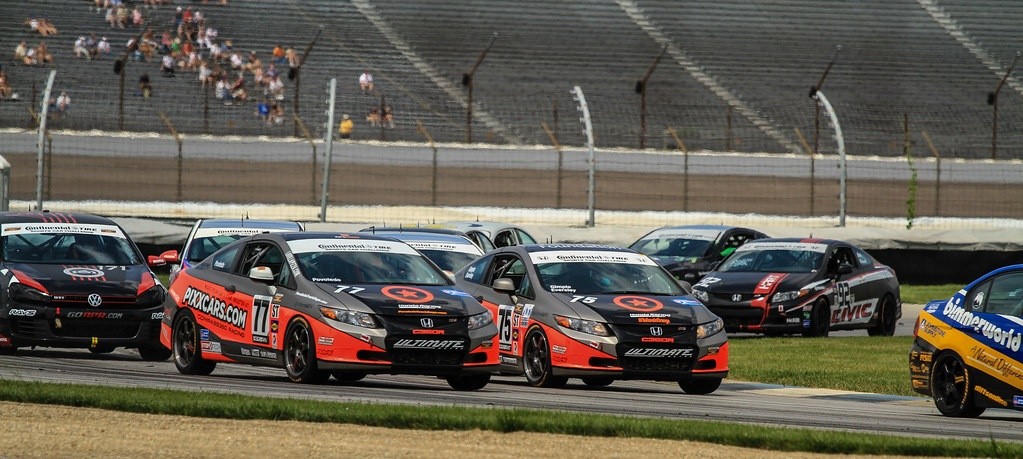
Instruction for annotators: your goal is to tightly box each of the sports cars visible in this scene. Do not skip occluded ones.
[175,217,306,272]
[692,236,905,341]
[437,218,537,255]
[906,261,1023,420]
[0,207,175,364]
[158,229,501,391]
[628,221,772,284]
[358,226,486,279]
[450,241,730,397]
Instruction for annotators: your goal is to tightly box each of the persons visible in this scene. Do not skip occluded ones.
[367,105,394,129]
[15,40,52,65]
[94,0,173,29]
[130,6,300,124]
[339,114,353,138]
[74,34,112,61]
[359,71,374,91]
[26,16,57,36]
[39,90,71,112]
[0,71,13,99]
